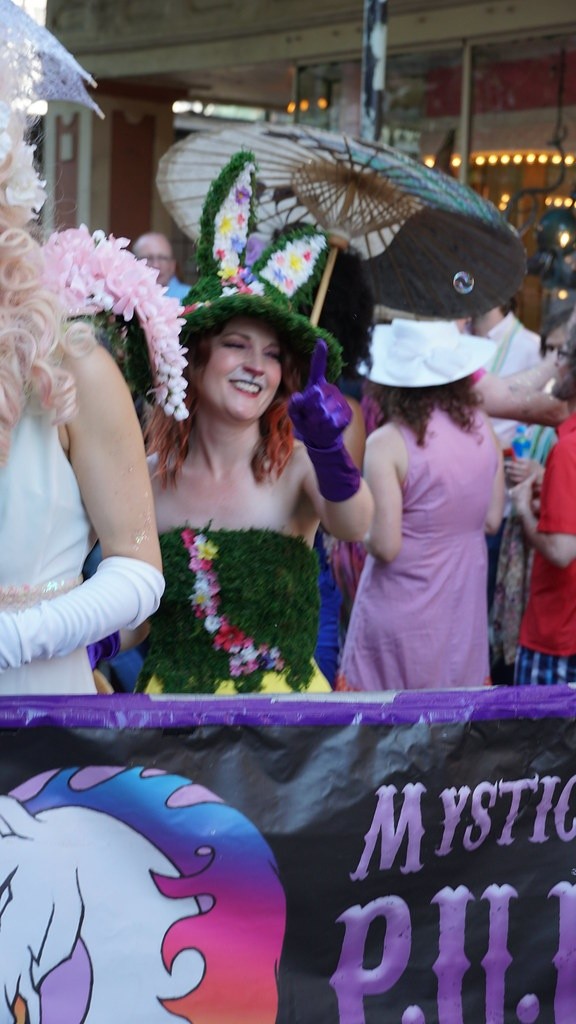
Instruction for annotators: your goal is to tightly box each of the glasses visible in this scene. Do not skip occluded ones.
[138,254,173,265]
[558,343,574,360]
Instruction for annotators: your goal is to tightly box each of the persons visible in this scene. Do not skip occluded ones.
[131,230,575,687]
[2,44,167,694]
[86,258,375,694]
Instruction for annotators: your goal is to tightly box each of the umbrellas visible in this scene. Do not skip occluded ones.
[154,121,528,328]
[0,1,106,122]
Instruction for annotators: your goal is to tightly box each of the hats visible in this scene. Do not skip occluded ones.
[367,317,497,387]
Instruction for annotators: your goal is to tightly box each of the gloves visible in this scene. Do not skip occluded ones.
[289,339,361,503]
